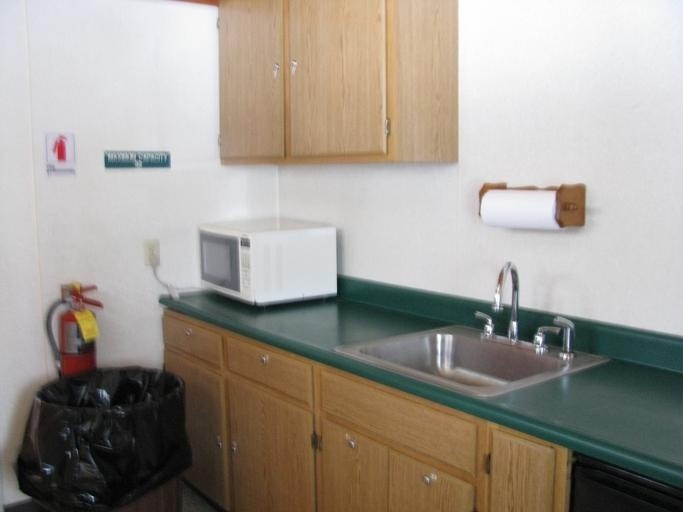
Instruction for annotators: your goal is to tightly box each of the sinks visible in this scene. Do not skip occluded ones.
[333,324,609,399]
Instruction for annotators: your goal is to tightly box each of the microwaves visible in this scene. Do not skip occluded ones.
[198,216,338,306]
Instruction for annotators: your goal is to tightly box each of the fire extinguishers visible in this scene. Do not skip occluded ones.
[46,282,104,380]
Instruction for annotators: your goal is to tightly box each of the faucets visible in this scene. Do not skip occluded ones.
[492,262,519,344]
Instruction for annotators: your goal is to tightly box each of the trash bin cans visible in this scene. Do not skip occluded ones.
[35,365,186,512]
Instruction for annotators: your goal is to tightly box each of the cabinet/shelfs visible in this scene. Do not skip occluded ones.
[164,312,317,510]
[481,417,571,511]
[319,359,477,511]
[217,2,459,167]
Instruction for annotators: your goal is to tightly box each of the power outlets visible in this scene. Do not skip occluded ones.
[142,237,160,266]
